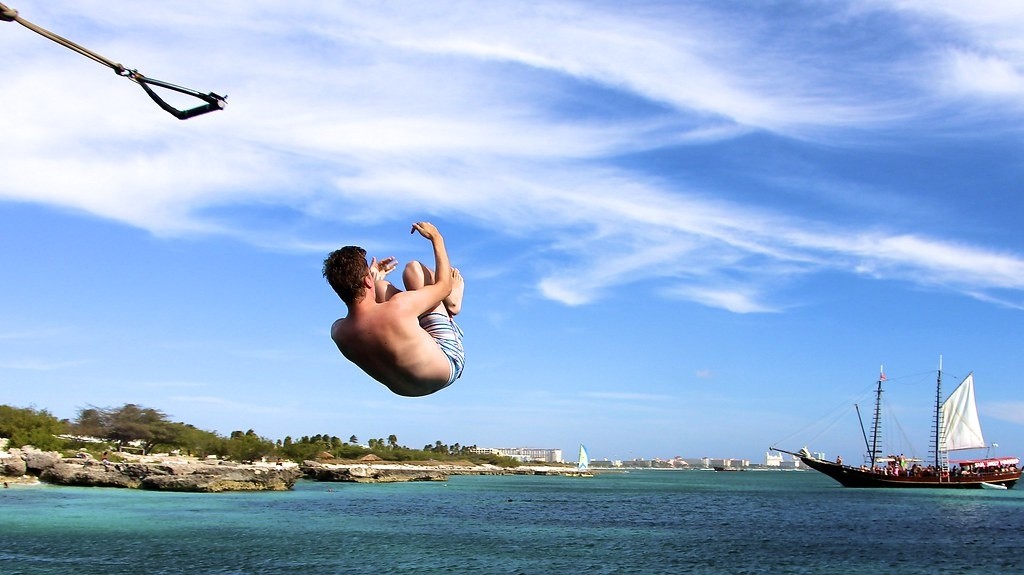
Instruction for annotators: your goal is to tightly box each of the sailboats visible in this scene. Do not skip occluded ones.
[566,443,593,477]
[769,354,1024,488]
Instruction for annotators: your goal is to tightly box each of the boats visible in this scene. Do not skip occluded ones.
[714,471,717,473]
[713,466,744,471]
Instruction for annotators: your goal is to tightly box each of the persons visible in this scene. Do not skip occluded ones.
[101,452,111,465]
[322,222,465,397]
[836,453,1017,477]
[3,482,9,488]
